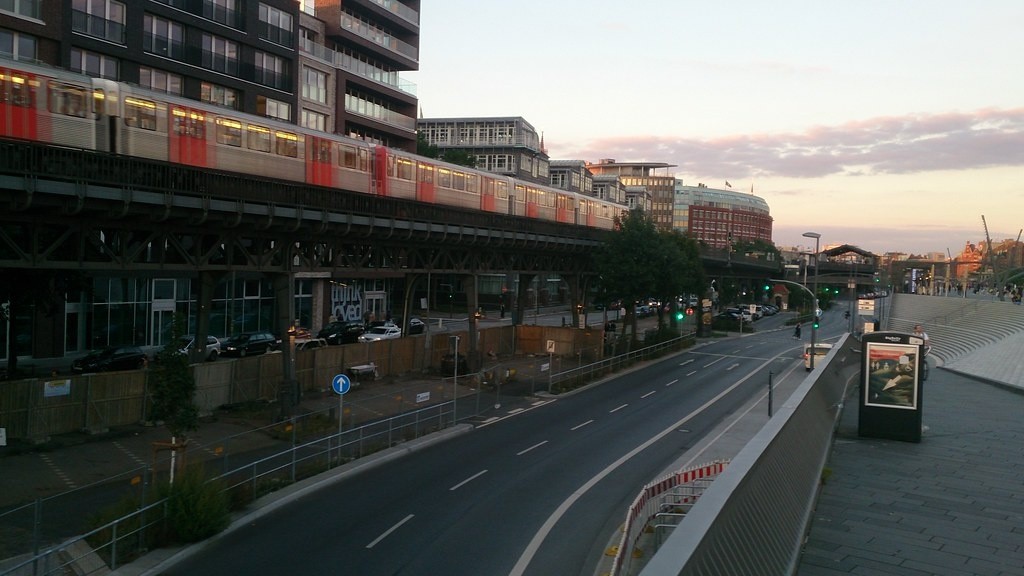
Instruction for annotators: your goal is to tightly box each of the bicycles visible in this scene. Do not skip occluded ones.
[920,345,933,381]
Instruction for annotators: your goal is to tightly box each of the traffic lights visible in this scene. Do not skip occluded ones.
[812,317,819,328]
[764,282,772,291]
[824,286,838,294]
[873,268,879,286]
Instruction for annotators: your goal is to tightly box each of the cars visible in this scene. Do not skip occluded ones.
[73,346,147,377]
[593,292,780,325]
[160,333,221,368]
[274,335,330,361]
[363,320,396,337]
[221,331,276,357]
[395,316,425,337]
[316,322,366,347]
[353,326,401,347]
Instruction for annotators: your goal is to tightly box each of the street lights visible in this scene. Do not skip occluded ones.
[770,232,822,371]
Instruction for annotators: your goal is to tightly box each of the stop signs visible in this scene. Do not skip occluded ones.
[502,287,507,293]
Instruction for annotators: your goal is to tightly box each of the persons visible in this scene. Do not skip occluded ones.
[913,325,930,349]
[792,322,802,341]
